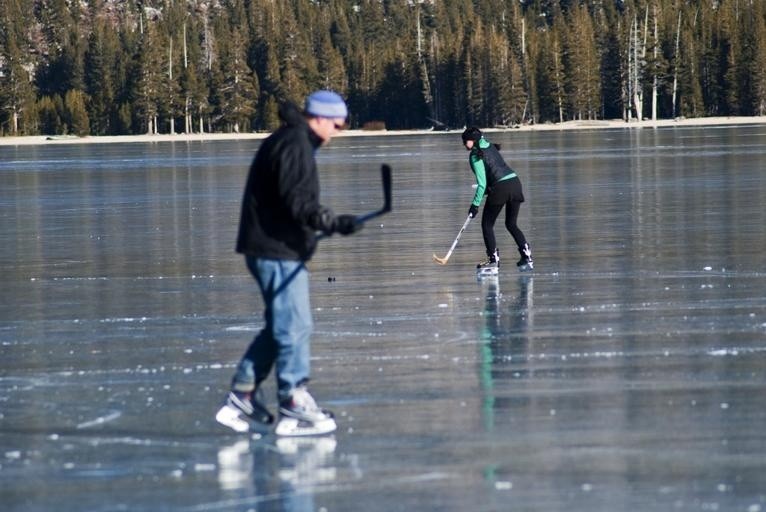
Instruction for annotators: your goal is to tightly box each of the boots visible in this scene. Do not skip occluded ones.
[477,248,500,268]
[517,243,534,266]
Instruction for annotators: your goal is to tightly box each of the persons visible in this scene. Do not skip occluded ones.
[461,122,535,271]
[215,439,338,511]
[479,274,533,494]
[212,89,365,440]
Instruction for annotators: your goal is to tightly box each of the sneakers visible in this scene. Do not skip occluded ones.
[278,391,333,422]
[227,391,273,424]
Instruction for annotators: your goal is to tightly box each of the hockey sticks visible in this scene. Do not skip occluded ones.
[431,213,473,265]
[316,163,392,240]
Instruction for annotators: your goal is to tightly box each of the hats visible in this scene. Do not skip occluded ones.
[462,127,481,139]
[303,91,348,118]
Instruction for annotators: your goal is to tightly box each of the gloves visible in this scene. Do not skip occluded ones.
[333,214,362,235]
[468,205,478,218]
